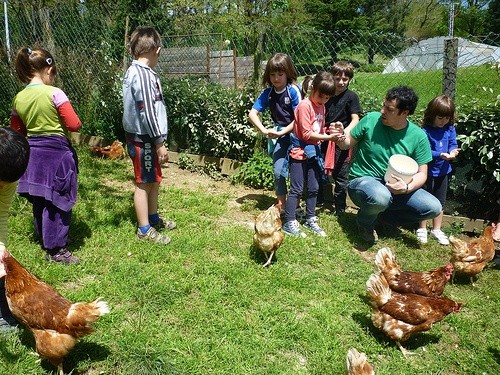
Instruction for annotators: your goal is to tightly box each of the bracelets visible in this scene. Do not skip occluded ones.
[406,184,408,192]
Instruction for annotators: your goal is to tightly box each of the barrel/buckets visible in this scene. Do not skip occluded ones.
[384,154,418,184]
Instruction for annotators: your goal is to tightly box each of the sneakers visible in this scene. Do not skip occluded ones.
[281,220,307,239]
[303,216,326,237]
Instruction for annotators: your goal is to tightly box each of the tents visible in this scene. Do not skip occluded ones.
[383,36,500,75]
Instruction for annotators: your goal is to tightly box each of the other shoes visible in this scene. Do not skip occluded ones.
[136,227,171,245]
[355,218,378,242]
[376,213,402,236]
[333,208,344,216]
[415,228,428,245]
[46,248,80,265]
[149,216,176,230]
[322,203,334,212]
[279,208,285,219]
[431,229,450,245]
[295,206,305,224]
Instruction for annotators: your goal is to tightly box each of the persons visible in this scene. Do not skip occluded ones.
[329,84,442,244]
[0,125,30,333]
[250,53,304,219]
[316,60,360,217]
[122,27,176,245]
[10,47,82,266]
[282,72,345,239]
[417,95,461,244]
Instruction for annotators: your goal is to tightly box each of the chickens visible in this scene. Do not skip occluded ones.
[0,252,111,375]
[374,246,453,295]
[253,201,285,268]
[366,269,464,359]
[93,139,125,161]
[448,222,496,288]
[345,343,375,375]
